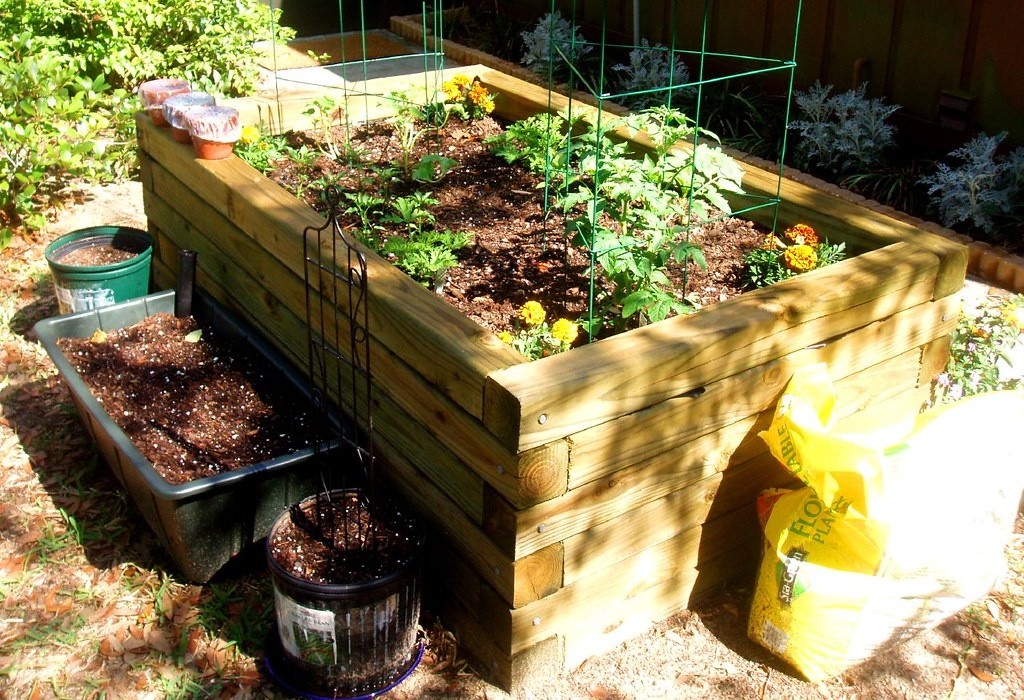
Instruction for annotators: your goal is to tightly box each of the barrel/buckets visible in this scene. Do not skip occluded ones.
[185,107,239,159]
[44,226,152,316]
[142,79,190,125]
[165,92,216,144]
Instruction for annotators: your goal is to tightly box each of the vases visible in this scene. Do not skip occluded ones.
[141,78,190,126]
[183,106,239,160]
[163,92,215,144]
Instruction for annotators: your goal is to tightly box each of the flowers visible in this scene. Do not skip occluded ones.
[408,73,500,122]
[742,224,847,292]
[917,286,1024,414]
[498,299,581,360]
